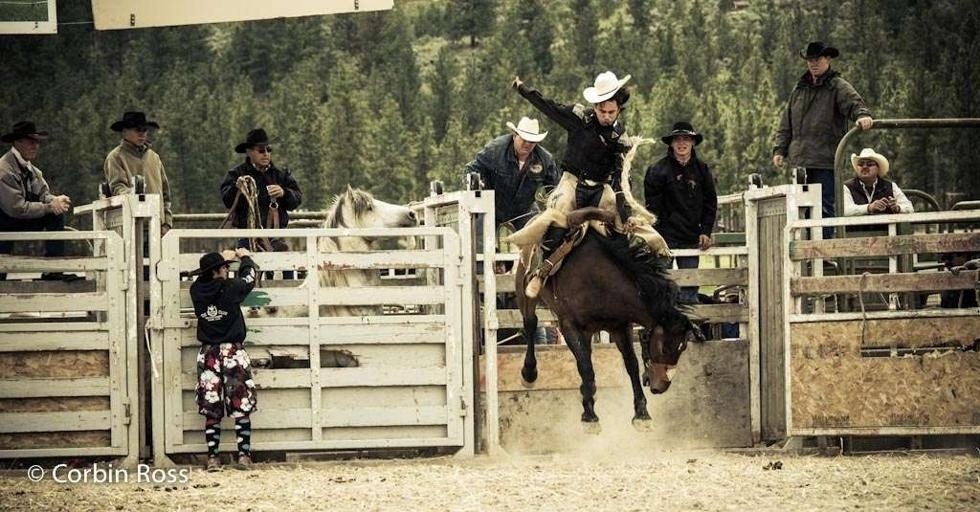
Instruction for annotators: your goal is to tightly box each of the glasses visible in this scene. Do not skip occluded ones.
[252,148,273,154]
[132,129,148,133]
[857,163,877,168]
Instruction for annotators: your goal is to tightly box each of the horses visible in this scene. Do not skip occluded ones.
[241,182,420,317]
[515,185,711,435]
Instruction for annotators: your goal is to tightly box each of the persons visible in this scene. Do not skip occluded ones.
[512,71,670,300]
[644,122,716,343]
[190,248,255,471]
[463,119,560,254]
[772,43,872,272]
[216,131,302,230]
[0,122,73,254]
[99,109,173,258]
[843,148,914,233]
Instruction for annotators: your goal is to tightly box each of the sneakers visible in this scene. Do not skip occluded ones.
[208,457,223,471]
[238,455,254,469]
[525,277,542,298]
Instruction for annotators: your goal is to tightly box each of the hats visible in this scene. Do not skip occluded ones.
[111,112,161,132]
[800,42,840,61]
[851,149,889,179]
[662,122,703,146]
[507,117,549,144]
[1,122,49,144]
[584,72,632,104]
[236,129,278,154]
[187,254,239,277]
